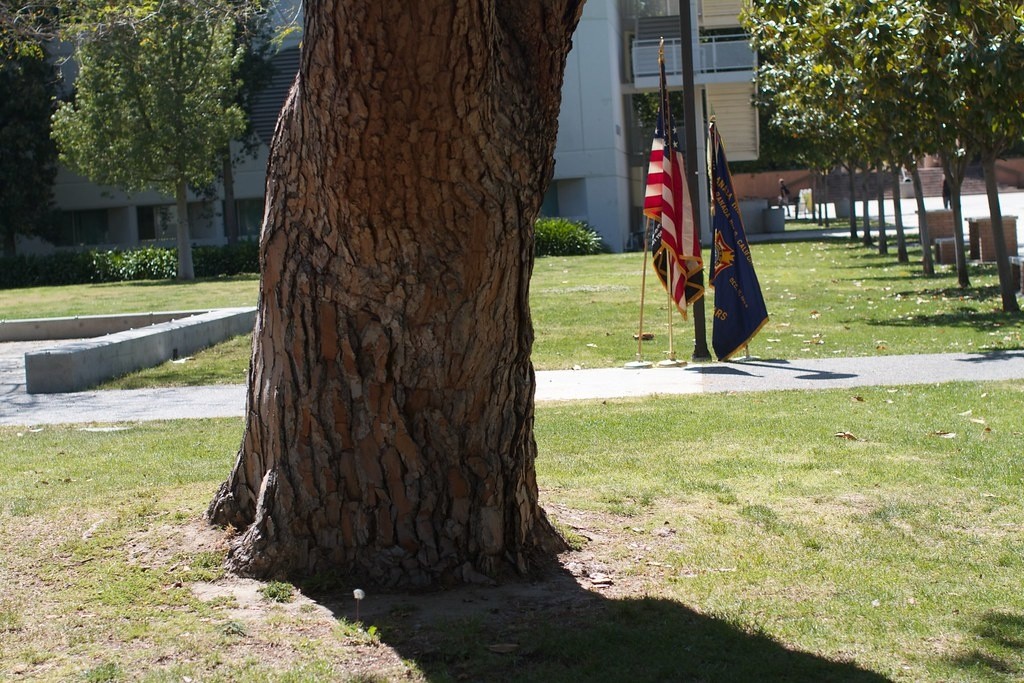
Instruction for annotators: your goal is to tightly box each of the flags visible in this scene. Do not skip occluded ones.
[707,116,769,361]
[643,57,705,322]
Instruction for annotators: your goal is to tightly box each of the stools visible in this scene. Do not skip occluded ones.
[934,237,957,263]
[1008,256,1024,295]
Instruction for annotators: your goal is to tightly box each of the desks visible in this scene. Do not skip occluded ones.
[916,209,957,247]
[964,216,1019,263]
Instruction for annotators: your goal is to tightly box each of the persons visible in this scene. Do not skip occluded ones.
[942,172,952,209]
[778,179,790,217]
